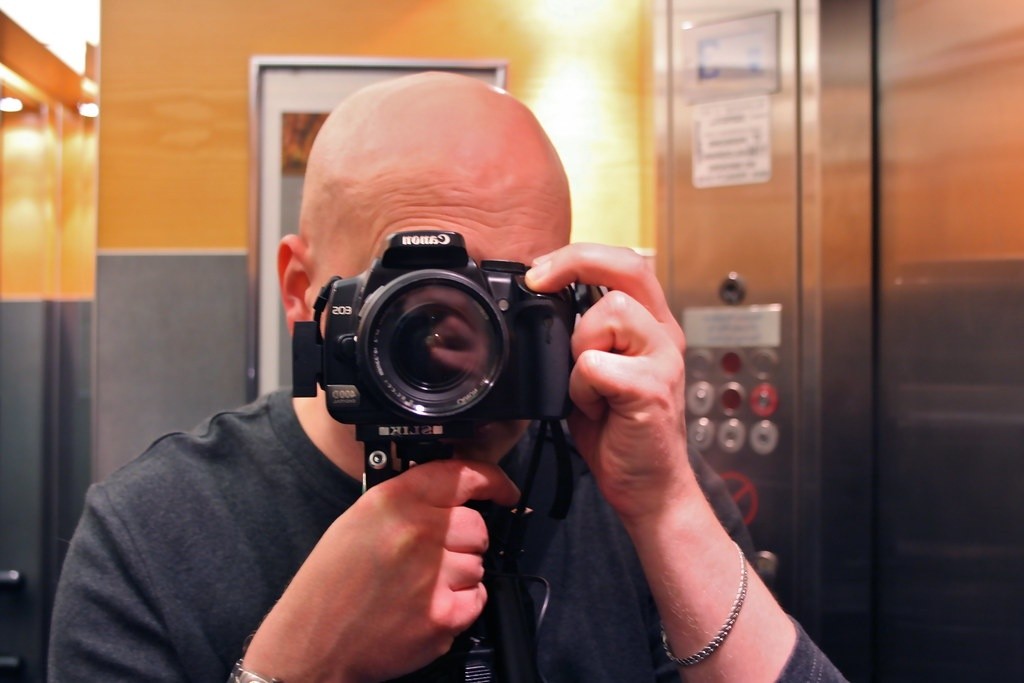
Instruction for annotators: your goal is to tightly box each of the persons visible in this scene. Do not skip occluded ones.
[48,72,855,683]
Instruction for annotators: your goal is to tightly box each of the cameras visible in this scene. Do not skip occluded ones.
[321,229,576,441]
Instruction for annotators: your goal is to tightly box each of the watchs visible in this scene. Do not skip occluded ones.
[230,658,282,683]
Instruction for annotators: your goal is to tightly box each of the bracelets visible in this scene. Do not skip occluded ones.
[661,540,747,667]
